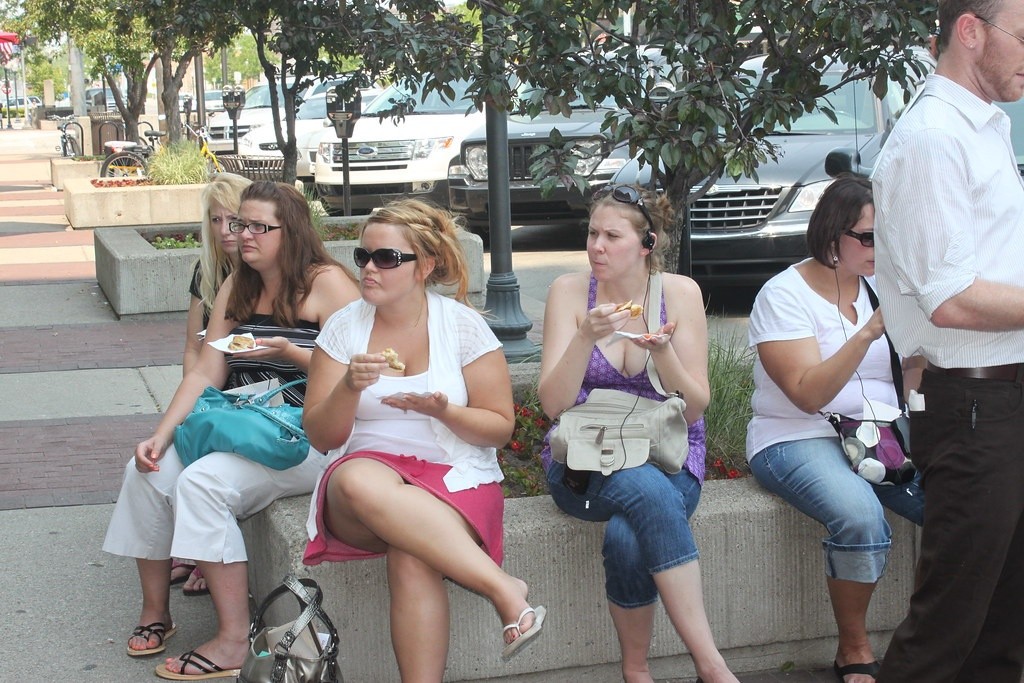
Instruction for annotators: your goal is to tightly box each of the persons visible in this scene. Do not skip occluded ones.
[0,102,4,131]
[302,198,547,683]
[124,172,363,680]
[871,1,1024,683]
[746,173,927,683]
[539,185,743,683]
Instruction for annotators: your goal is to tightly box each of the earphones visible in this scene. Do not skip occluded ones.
[643,231,655,254]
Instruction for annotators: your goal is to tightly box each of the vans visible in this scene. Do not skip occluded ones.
[4,96,43,109]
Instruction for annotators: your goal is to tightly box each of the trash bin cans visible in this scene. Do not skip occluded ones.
[88,111,124,156]
[103,141,137,166]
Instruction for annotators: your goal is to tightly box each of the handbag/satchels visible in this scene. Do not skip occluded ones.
[550,385,689,477]
[240,575,343,683]
[825,412,918,486]
[173,378,311,471]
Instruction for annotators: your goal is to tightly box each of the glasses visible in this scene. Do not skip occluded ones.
[593,186,655,233]
[353,246,420,268]
[229,218,286,234]
[842,226,874,248]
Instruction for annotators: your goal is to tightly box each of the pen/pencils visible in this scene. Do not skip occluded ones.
[972,400,978,430]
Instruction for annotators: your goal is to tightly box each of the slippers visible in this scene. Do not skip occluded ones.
[126,623,177,655]
[500,604,547,661]
[183,565,210,595]
[169,560,195,584]
[833,656,881,682]
[156,651,246,680]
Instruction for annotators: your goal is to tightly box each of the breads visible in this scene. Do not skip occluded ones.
[228,336,254,351]
[615,300,643,318]
[379,348,406,370]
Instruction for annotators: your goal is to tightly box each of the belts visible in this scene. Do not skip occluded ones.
[926,359,1024,385]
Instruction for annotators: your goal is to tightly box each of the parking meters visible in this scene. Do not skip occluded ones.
[222,85,246,155]
[325,83,362,217]
[182,95,194,142]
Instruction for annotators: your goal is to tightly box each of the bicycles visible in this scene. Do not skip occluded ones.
[100,111,228,177]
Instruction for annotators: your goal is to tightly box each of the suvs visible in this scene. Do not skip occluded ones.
[607,42,941,320]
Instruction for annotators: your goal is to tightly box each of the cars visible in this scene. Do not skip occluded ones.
[447,22,798,249]
[237,86,385,182]
[315,61,539,218]
[34,96,95,119]
[85,88,127,113]
[990,91,1024,184]
[158,89,230,114]
